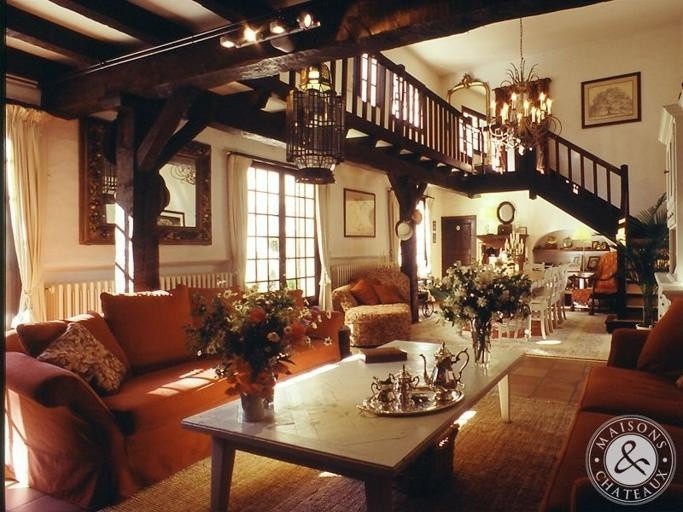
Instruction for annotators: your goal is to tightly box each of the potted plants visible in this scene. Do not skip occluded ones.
[610,191,674,331]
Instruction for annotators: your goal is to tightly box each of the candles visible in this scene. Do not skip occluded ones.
[502,230,526,256]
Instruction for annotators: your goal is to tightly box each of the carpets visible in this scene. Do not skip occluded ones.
[412,305,614,362]
[92,389,584,511]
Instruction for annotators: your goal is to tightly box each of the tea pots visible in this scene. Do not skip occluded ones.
[370,363,420,407]
[419,339,469,404]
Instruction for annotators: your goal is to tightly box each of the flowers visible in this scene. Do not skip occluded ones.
[424,255,534,326]
[187,279,332,398]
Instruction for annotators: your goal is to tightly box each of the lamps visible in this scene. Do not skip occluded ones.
[219,5,324,61]
[484,15,564,152]
[283,59,352,188]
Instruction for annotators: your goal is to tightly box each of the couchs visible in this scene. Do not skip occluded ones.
[2,300,342,512]
[537,295,682,512]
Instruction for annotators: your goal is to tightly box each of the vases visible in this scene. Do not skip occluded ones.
[469,322,495,367]
[239,375,278,423]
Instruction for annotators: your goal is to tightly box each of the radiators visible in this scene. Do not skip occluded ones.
[331,261,387,290]
[49,271,237,322]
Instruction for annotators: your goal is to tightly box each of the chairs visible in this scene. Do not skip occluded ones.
[499,260,576,341]
[568,250,622,315]
[332,267,410,347]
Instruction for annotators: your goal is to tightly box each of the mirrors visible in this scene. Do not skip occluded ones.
[78,115,213,247]
[497,201,515,224]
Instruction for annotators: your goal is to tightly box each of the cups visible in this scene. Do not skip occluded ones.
[591,240,608,250]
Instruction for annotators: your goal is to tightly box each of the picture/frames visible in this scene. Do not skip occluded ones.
[579,69,644,131]
[585,254,602,271]
[157,210,185,227]
[341,187,378,239]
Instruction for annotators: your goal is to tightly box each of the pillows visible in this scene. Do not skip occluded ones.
[634,294,682,370]
[95,286,197,368]
[258,288,304,308]
[350,276,380,306]
[13,310,130,384]
[36,321,131,394]
[176,280,243,312]
[371,282,403,304]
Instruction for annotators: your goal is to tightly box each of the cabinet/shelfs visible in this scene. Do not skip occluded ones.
[655,100,682,323]
[531,242,616,293]
[475,233,528,275]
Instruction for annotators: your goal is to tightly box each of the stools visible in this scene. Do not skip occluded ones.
[344,302,410,351]
